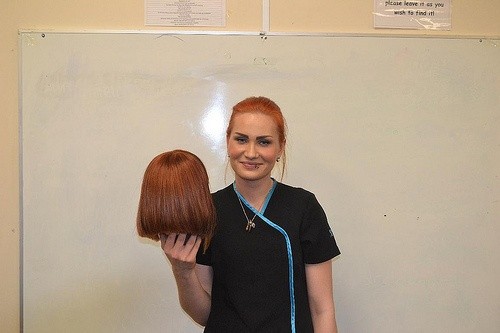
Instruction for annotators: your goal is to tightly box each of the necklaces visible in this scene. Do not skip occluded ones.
[234,182,274,231]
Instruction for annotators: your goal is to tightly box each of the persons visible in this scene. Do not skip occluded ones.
[156,96,341,332]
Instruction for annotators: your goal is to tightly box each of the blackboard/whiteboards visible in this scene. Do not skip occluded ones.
[16,31,500,333]
